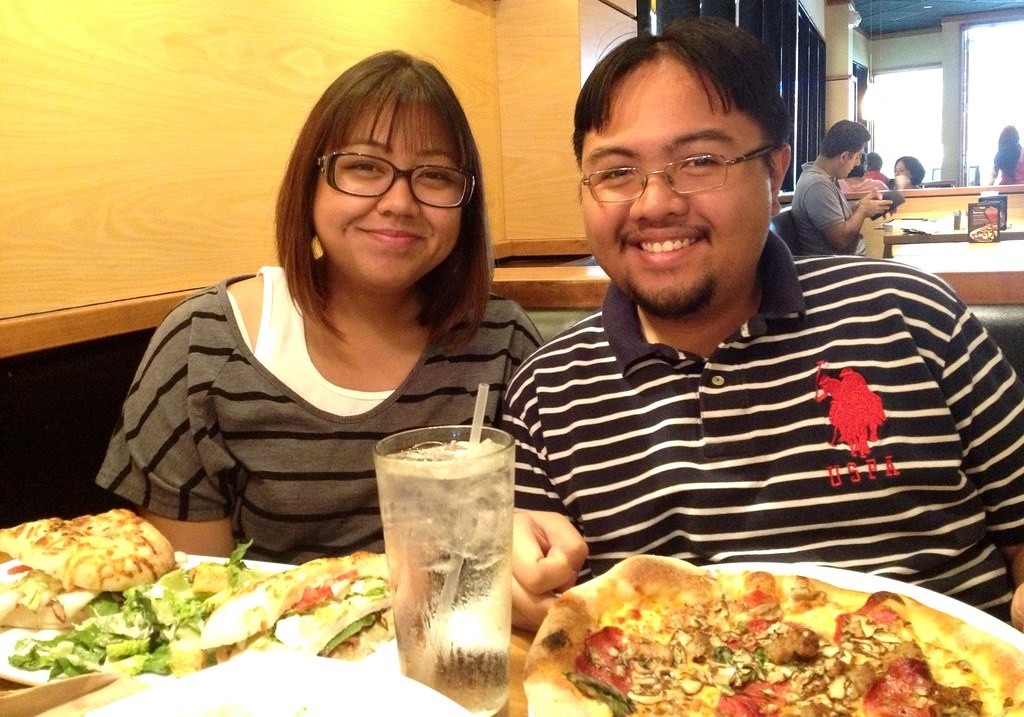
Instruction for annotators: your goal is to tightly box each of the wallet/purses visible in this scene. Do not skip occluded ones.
[869,190,904,220]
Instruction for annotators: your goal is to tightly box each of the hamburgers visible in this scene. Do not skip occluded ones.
[202,550,394,662]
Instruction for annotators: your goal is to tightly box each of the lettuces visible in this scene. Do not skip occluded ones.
[8,539,270,677]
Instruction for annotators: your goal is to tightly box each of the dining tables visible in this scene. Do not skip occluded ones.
[881,214,1024,260]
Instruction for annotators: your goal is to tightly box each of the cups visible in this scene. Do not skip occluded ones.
[372,425,515,717]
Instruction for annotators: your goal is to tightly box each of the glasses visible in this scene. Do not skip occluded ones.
[583,145,776,202]
[317,151,477,209]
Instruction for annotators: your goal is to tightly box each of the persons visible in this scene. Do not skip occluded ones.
[500,16,1024,633]
[894,156,925,190]
[94,49,545,566]
[988,125,1024,195]
[862,153,889,185]
[790,120,893,256]
[837,152,890,192]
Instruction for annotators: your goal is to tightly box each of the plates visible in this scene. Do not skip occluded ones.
[970,230,992,242]
[0,558,60,686]
[697,562,1024,653]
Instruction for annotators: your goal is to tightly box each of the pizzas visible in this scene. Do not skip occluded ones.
[520,554,1024,717]
[0,565,100,630]
[0,509,177,591]
